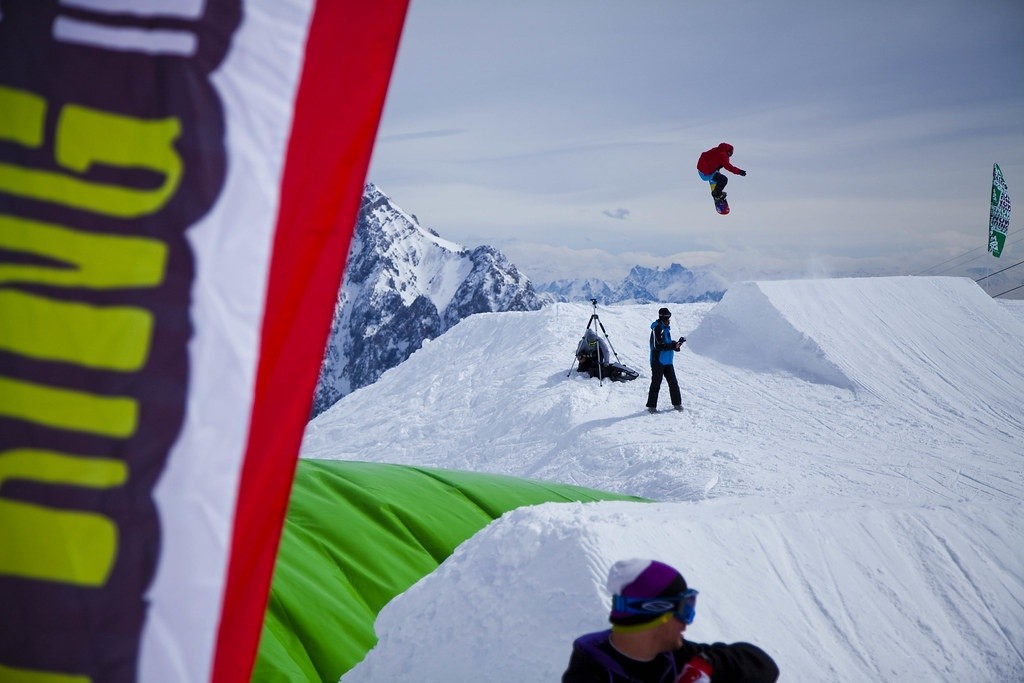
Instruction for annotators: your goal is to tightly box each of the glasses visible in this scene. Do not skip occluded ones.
[674,588,698,625]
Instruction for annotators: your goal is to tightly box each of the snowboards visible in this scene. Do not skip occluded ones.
[709,179,731,215]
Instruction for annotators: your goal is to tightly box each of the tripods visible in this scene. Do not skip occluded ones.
[567,299,621,387]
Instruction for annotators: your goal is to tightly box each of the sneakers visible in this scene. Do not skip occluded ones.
[712,190,727,200]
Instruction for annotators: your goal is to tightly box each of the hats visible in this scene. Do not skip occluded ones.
[607,559,687,624]
[659,307,671,319]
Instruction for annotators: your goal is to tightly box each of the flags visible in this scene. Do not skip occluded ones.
[988,163,1011,258]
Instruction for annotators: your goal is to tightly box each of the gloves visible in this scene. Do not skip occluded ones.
[739,170,746,177]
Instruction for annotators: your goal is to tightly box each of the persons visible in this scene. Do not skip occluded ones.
[697,142,746,198]
[561,557,779,682]
[645,307,684,413]
[576,329,610,372]
